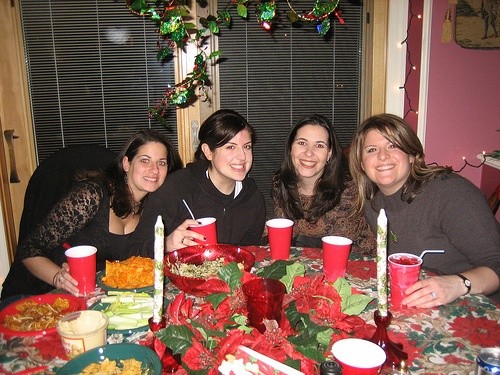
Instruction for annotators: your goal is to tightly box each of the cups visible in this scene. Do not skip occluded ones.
[266,218,295,261]
[189,217,217,247]
[388,253,423,305]
[332,337,386,375]
[321,235,352,284]
[64,245,97,296]
[241,277,286,334]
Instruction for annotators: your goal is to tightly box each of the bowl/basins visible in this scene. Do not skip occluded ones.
[56,343,162,375]
[163,243,255,298]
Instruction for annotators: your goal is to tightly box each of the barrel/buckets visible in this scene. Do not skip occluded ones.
[56,310,108,362]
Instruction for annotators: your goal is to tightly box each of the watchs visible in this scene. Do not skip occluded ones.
[456,273,472,296]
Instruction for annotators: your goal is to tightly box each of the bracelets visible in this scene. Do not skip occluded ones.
[52,271,60,285]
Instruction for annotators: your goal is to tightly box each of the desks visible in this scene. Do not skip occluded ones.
[0,248,500,375]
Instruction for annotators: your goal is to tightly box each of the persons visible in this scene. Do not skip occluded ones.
[347,114,500,308]
[0,128,183,311]
[271,114,377,258]
[131,109,267,256]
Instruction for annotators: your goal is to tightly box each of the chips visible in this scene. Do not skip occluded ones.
[80,356,147,375]
[102,255,156,288]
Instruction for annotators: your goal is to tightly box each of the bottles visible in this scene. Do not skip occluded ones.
[320,360,341,375]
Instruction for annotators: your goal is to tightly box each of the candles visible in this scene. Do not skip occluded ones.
[376,209,387,317]
[152,215,164,323]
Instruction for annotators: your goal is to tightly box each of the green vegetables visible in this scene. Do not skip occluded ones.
[100,291,153,330]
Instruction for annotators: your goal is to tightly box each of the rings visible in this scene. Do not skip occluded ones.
[59,277,63,282]
[431,292,436,300]
[181,237,185,243]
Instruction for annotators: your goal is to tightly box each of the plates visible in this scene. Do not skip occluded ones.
[97,270,170,293]
[0,294,87,336]
[88,296,169,334]
